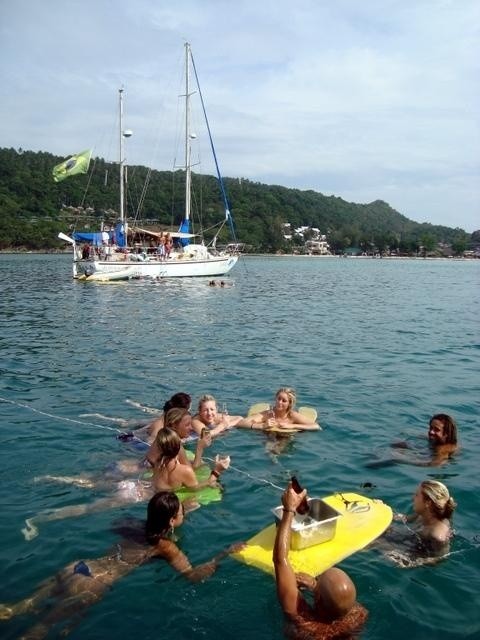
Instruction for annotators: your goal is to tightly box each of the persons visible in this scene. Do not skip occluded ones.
[19,427,231,540]
[235,388,322,431]
[82,225,173,261]
[1,491,247,639]
[366,413,460,467]
[30,407,213,488]
[77,394,244,437]
[365,479,458,569]
[116,392,192,443]
[273,481,369,639]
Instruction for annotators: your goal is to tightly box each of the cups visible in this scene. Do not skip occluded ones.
[219,453,226,460]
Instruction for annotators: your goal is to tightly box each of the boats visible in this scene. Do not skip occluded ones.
[75,265,143,283]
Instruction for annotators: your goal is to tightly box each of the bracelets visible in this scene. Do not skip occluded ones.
[283,509,297,516]
[251,424,254,429]
[209,469,219,478]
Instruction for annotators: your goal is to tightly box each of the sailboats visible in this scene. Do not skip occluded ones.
[58,42,242,287]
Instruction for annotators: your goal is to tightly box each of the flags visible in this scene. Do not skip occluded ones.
[52,148,93,183]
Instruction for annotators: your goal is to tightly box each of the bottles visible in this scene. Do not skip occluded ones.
[267,405,275,420]
[291,475,310,516]
[222,402,228,419]
